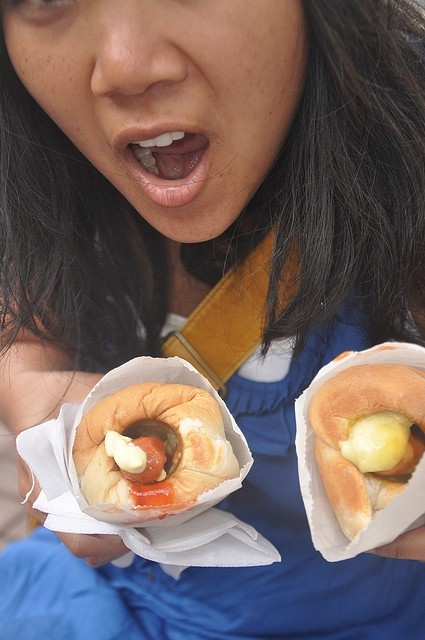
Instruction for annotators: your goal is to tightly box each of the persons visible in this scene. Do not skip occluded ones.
[0,0,424,640]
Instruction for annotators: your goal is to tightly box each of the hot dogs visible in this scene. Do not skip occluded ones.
[307,360,425,545]
[70,380,241,522]
